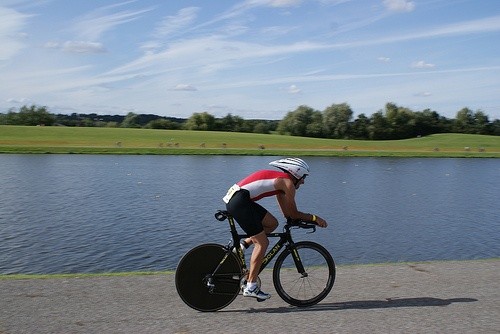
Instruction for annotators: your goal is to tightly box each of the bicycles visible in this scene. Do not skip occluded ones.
[175,209,336,311]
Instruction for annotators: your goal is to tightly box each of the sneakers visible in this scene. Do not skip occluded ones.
[242,286,271,300]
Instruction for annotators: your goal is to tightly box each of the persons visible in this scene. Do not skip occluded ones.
[222,156,329,301]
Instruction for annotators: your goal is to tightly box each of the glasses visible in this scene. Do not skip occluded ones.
[302,174,306,179]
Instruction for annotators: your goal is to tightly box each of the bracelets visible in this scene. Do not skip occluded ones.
[312,214,317,222]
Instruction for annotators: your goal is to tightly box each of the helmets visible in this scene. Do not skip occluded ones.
[268,157,311,180]
